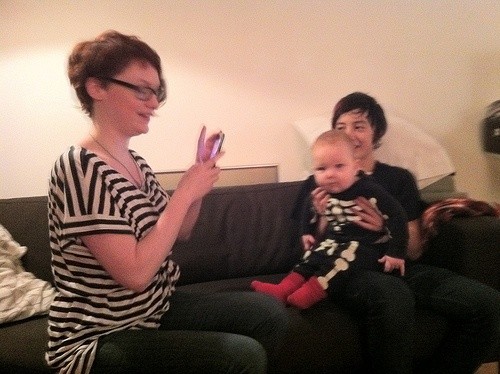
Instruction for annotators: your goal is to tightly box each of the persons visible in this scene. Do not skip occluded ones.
[290,91,500,374]
[251,129,408,308]
[46,30,289,374]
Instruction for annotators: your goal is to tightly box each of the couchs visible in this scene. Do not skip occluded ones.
[0,181,500,374]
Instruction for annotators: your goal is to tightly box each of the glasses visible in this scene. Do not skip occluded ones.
[108,78,166,103]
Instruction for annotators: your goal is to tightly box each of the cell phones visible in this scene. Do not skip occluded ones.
[210,133,225,159]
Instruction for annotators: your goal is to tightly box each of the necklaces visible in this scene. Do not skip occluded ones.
[87,130,147,192]
